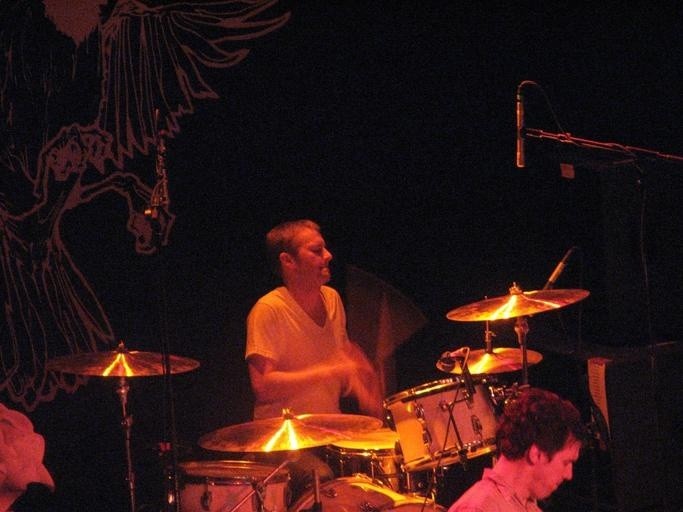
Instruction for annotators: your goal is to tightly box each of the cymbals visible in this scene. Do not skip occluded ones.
[436,348,543,376]
[44,351,199,379]
[197,414,383,453]
[446,288,591,323]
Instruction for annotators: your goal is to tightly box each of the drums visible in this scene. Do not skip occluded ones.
[173,459,291,511]
[383,373,500,472]
[319,430,437,501]
[285,474,446,512]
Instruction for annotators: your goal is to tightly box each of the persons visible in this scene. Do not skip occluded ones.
[245,219,383,421]
[447,389,584,512]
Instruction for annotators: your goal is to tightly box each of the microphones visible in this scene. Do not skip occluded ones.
[542,248,572,288]
[457,353,476,394]
[517,86,525,169]
